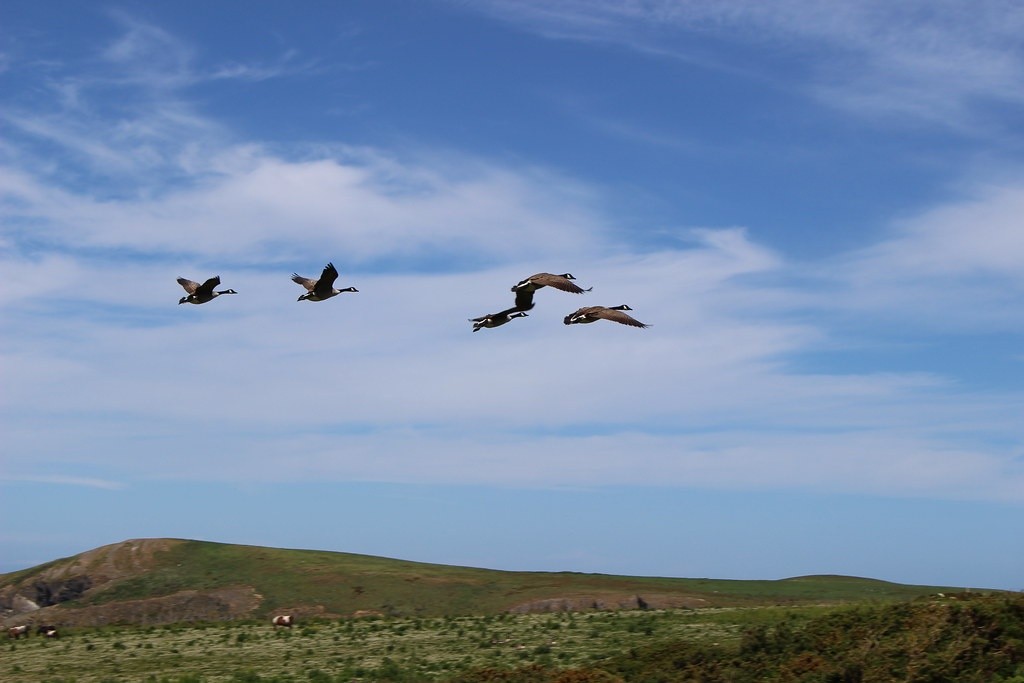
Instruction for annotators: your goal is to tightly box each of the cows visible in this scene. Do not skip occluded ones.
[8,625,32,640]
[272,615,295,631]
[36,624,59,640]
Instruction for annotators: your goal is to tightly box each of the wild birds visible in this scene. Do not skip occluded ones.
[468,303,536,333]
[563,304,653,330]
[291,262,360,303]
[176,274,238,305]
[511,272,593,309]
